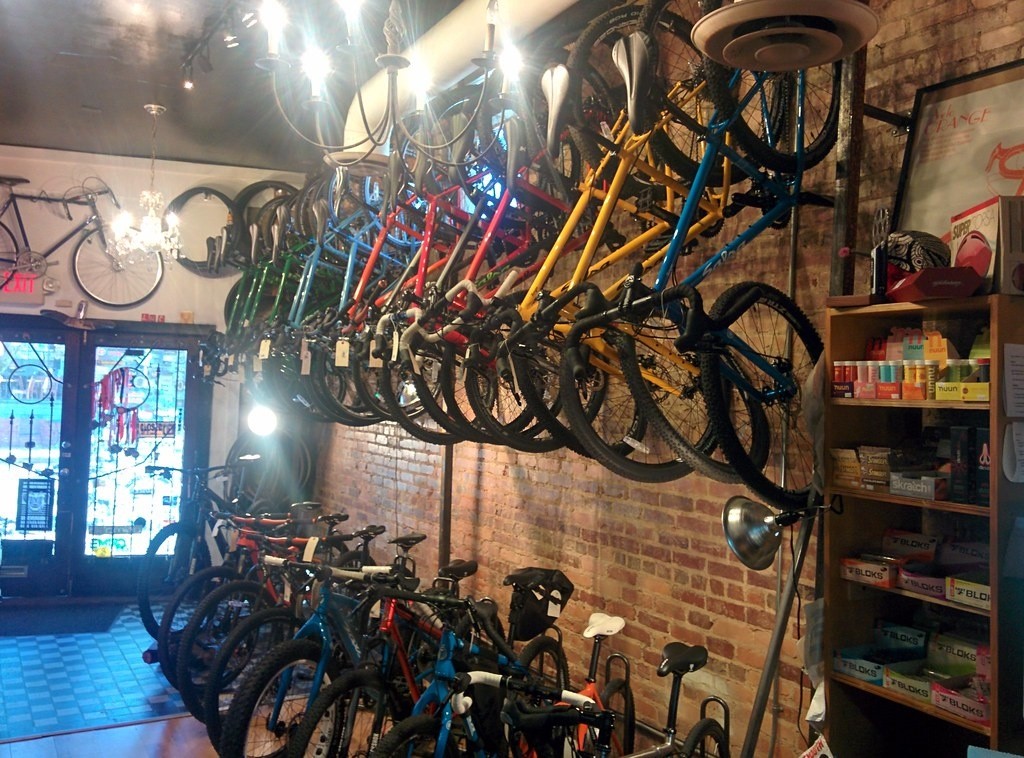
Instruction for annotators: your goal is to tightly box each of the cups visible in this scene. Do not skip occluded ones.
[834,358,990,400]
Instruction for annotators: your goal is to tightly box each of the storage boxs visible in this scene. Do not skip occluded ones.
[949,427,990,507]
[833,601,990,727]
[829,407,990,507]
[830,445,950,501]
[839,508,991,611]
[935,381,990,401]
[950,195,1024,295]
[830,381,927,400]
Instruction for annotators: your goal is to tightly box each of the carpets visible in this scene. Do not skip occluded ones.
[2,603,328,744]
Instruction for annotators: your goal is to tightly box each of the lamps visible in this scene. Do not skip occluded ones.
[197,39,213,73]
[183,58,193,89]
[104,103,187,268]
[220,15,239,49]
[0,270,60,307]
[257,0,518,166]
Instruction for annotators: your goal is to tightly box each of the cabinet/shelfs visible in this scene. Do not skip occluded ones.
[824,294,1024,758]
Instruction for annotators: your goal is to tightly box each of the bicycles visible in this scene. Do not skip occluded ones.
[0,174,165,309]
[138,0,862,757]
